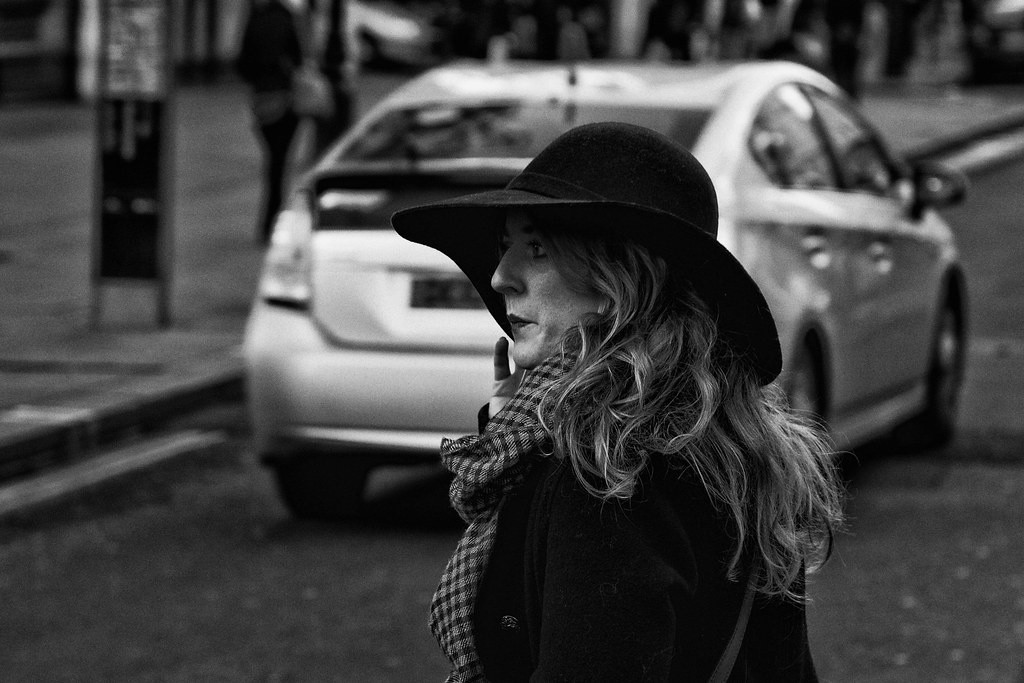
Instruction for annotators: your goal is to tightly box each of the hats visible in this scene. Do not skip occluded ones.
[389,121,783,386]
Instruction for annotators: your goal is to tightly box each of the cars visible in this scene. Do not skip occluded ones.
[243,57,971,525]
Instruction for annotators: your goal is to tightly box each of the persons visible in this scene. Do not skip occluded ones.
[235,0,1001,249]
[389,120,843,683]
